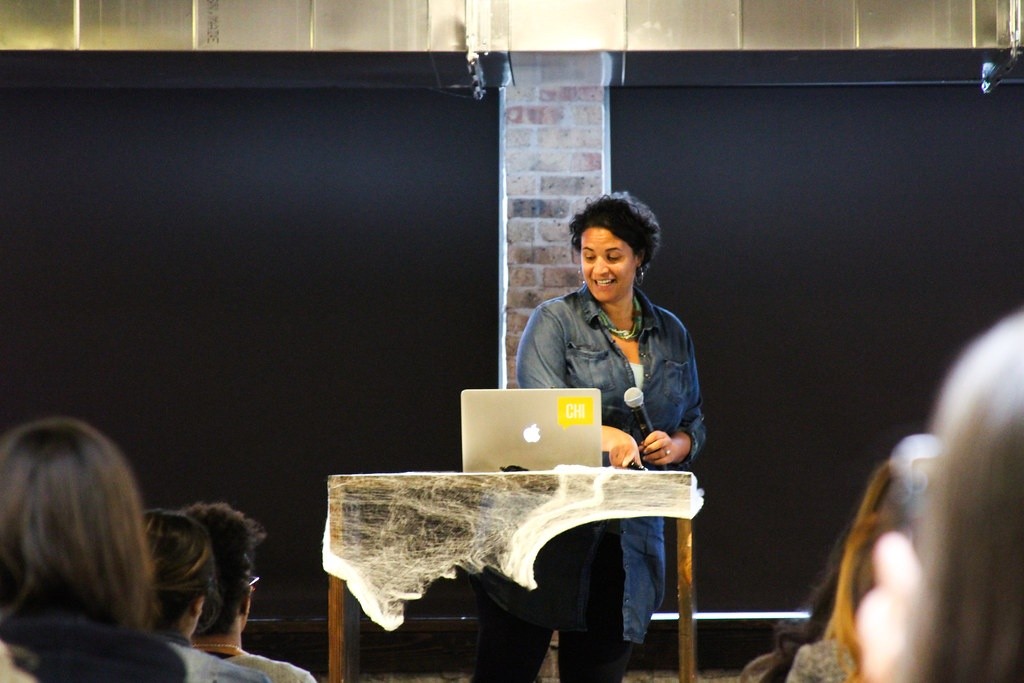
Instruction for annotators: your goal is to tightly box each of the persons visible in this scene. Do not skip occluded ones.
[737,307,1024,683]
[469,193,707,683]
[0,418,317,683]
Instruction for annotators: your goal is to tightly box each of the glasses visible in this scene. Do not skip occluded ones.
[248,575,260,586]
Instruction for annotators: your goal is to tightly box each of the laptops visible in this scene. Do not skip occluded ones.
[460,387,602,474]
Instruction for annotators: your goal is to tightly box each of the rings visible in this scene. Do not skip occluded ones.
[664,447,671,456]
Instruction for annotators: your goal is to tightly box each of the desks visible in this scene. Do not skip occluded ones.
[321,470,706,683]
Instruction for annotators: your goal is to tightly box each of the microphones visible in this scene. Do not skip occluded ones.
[624,387,668,471]
